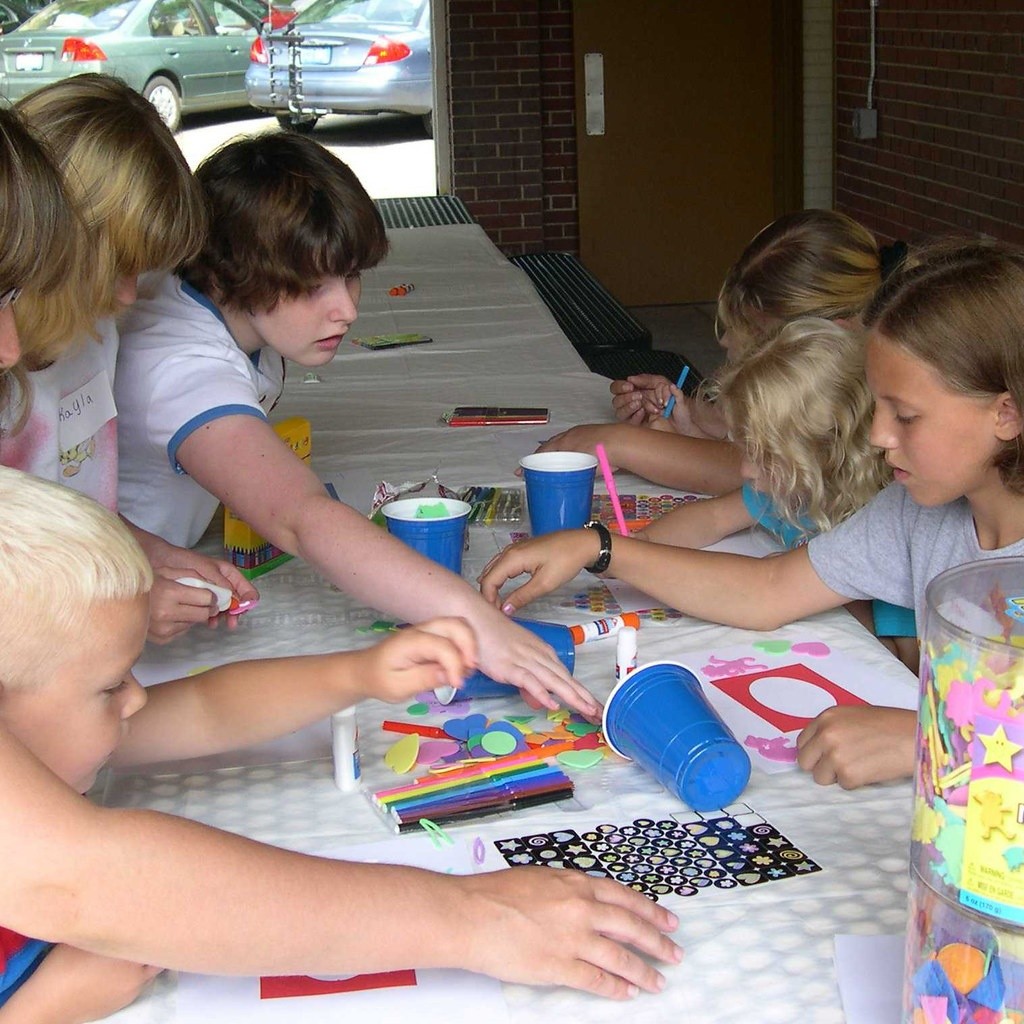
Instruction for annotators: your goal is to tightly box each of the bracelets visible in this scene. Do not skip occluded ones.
[583,520,610,573]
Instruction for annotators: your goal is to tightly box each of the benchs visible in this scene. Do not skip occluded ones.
[507,251,716,404]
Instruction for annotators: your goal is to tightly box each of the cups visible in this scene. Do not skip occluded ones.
[433,614,575,707]
[601,660,751,812]
[380,497,472,576]
[519,451,600,537]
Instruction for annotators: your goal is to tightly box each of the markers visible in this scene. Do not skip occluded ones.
[461,486,524,527]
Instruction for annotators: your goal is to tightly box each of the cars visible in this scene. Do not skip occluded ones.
[0,0,313,135]
[244,1,435,140]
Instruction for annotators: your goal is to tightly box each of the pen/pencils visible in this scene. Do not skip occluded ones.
[372,754,576,834]
[663,366,689,418]
[448,406,550,427]
[413,740,574,786]
[596,443,629,538]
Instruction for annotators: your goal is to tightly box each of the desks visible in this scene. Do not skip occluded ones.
[65,194,922,1024]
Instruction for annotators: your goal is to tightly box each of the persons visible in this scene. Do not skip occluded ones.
[476,236,1023,792]
[116,135,606,724]
[0,105,681,1024]
[0,72,260,646]
[515,209,920,496]
[598,317,920,673]
